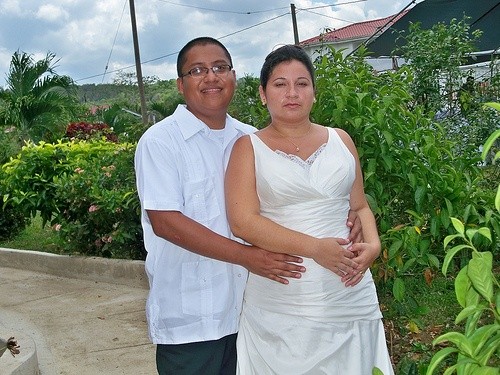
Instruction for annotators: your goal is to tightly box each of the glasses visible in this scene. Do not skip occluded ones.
[182,65,231,77]
